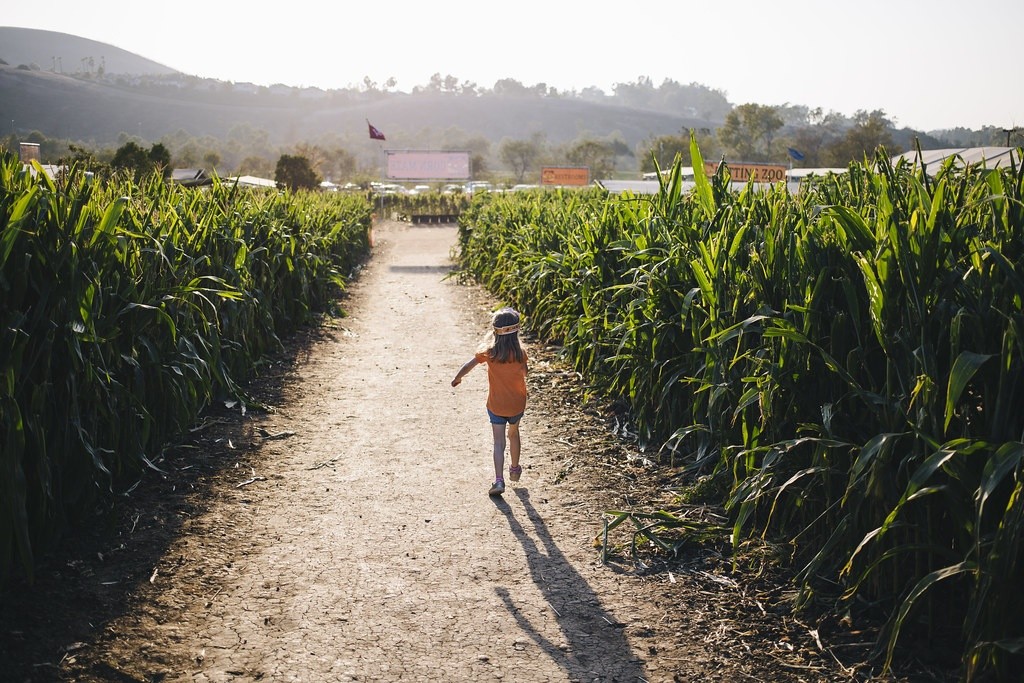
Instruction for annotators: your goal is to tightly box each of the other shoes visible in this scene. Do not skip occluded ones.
[509,465,521,481]
[488,481,505,495]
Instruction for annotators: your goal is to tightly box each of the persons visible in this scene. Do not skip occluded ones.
[451,307,529,495]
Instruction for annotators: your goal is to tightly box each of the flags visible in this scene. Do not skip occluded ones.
[369,125,386,140]
